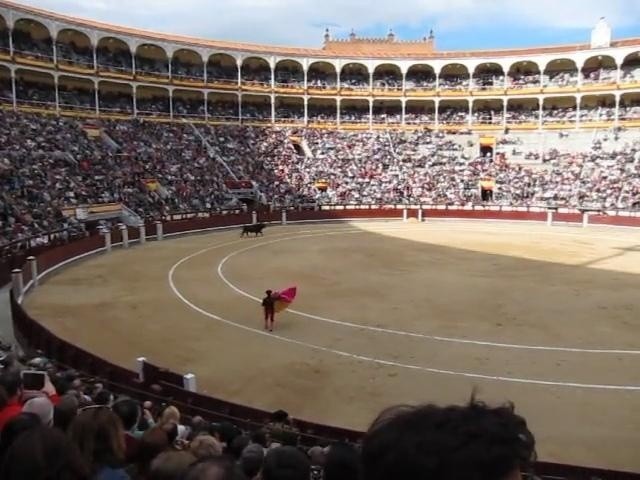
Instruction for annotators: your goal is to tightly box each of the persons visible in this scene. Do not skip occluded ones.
[1,105,640,257]
[0,342,362,479]
[1,28,640,125]
[353,382,538,480]
[262,290,281,332]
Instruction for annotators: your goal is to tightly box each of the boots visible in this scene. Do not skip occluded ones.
[265,320,272,332]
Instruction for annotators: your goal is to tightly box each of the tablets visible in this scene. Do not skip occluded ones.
[20,370,48,392]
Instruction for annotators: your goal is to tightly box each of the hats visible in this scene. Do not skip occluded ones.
[266,290,272,294]
[25,397,55,429]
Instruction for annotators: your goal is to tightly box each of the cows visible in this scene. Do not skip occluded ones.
[240,222,267,237]
